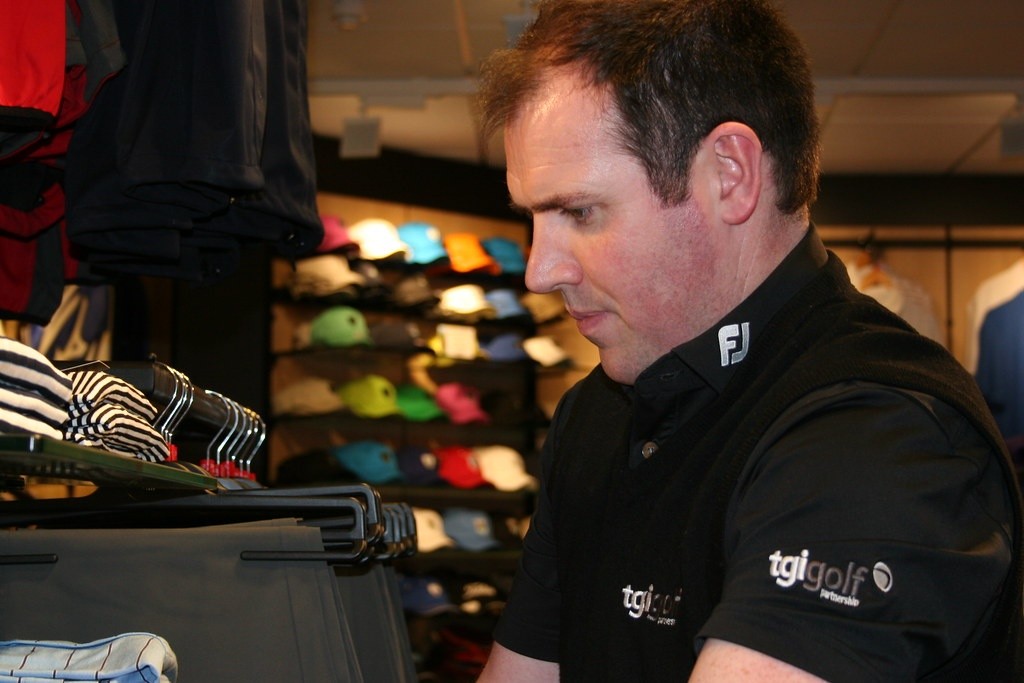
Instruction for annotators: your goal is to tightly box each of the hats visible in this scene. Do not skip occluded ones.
[269,214,573,621]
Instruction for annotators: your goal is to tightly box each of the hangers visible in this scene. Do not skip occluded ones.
[845,233,922,295]
[0,362,418,577]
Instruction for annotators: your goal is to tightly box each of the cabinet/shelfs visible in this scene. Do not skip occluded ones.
[271,259,572,620]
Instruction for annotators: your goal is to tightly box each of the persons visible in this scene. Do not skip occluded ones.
[476,0,1024,683]
